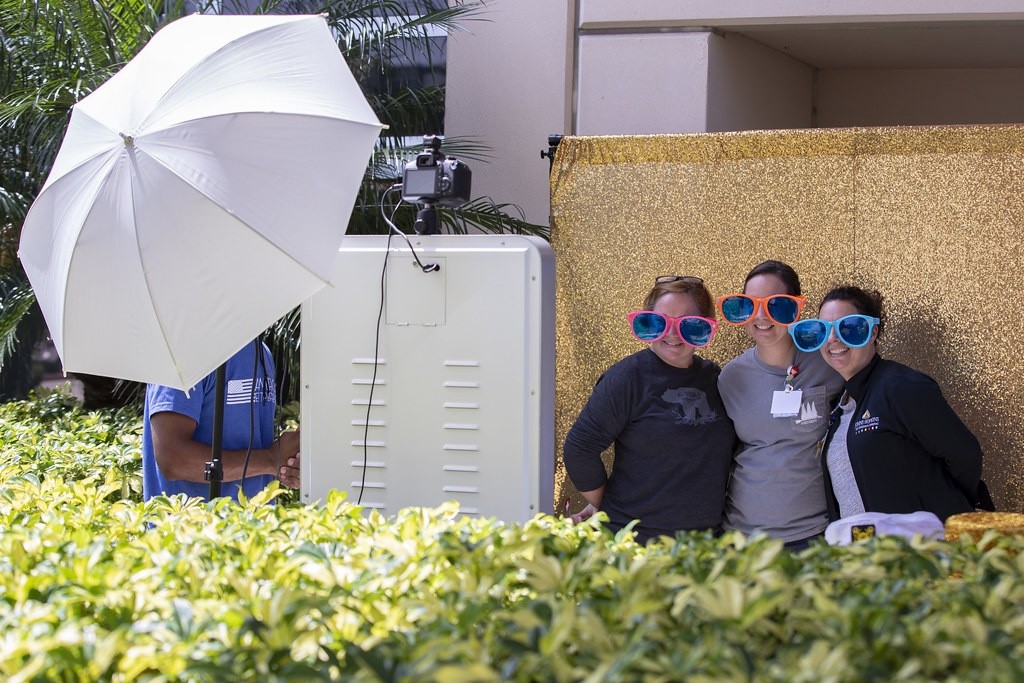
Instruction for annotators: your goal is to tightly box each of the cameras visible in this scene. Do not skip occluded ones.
[392,135,472,235]
[540,134,563,175]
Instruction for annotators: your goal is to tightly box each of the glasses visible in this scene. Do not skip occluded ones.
[655,275,704,285]
[715,293,807,326]
[787,315,882,353]
[628,310,718,348]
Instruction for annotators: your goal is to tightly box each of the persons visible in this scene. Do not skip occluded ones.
[561,277,739,546]
[815,282,999,543]
[139,338,301,506]
[712,261,845,546]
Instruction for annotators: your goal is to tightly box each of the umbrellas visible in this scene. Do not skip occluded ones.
[14,17,390,508]
